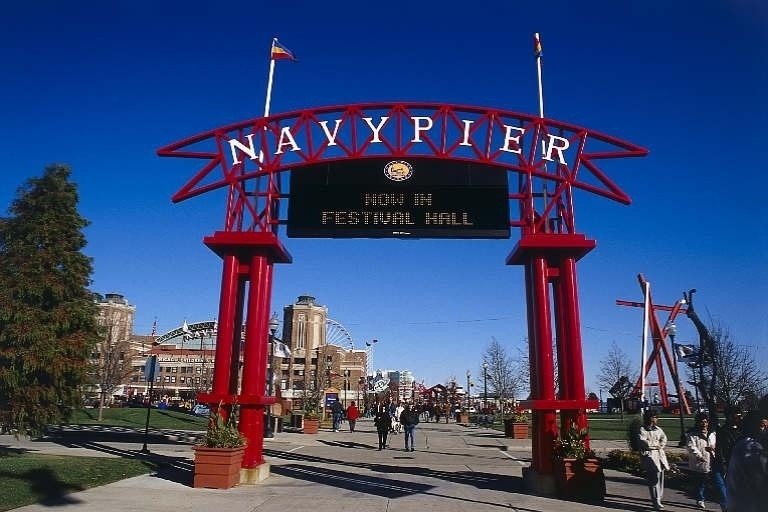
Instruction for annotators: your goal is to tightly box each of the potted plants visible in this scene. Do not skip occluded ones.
[550,417,606,500]
[502,414,530,440]
[190,394,250,490]
[303,408,322,434]
[453,406,471,424]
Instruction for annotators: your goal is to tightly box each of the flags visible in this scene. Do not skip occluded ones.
[534,34,543,64]
[271,39,303,64]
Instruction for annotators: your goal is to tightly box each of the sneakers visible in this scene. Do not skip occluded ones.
[654,501,664,508]
[697,500,706,509]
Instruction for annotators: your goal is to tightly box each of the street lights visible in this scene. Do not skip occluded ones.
[356,375,362,415]
[664,317,688,446]
[262,311,283,437]
[481,358,490,417]
[465,368,473,417]
[344,367,349,419]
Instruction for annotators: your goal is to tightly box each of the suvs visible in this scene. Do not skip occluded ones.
[461,406,476,414]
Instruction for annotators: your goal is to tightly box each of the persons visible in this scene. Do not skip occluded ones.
[113,389,210,416]
[331,396,451,450]
[713,406,746,484]
[729,414,768,512]
[686,413,728,511]
[637,408,670,508]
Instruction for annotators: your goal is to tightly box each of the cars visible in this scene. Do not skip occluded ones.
[694,405,748,419]
[74,389,210,417]
